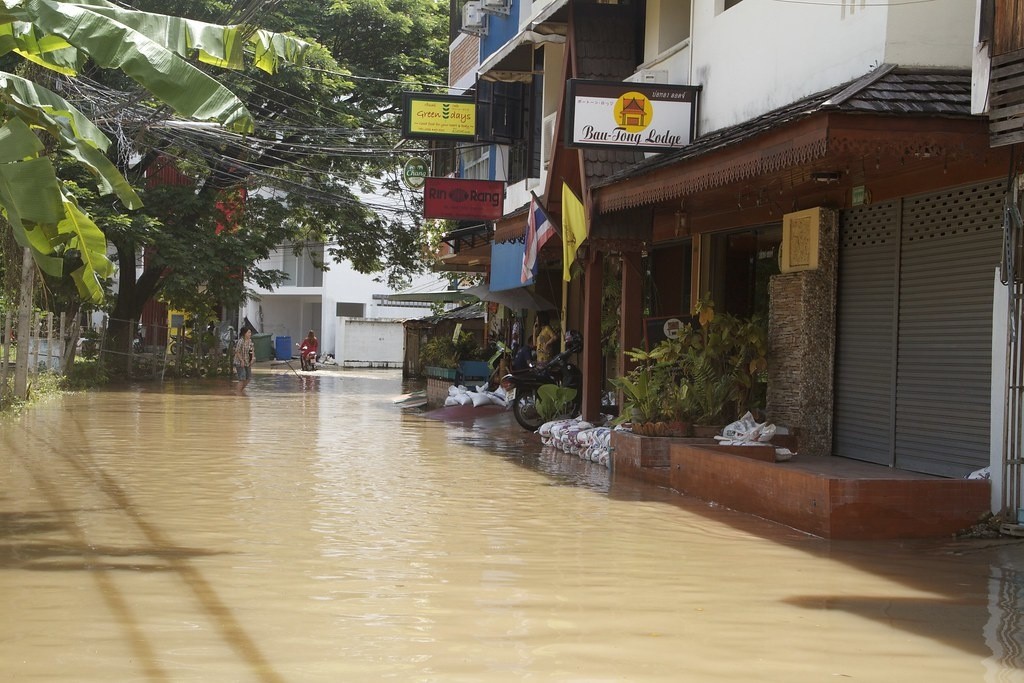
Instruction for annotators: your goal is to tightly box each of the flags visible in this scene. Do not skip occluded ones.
[562,182,586,281]
[521,197,556,284]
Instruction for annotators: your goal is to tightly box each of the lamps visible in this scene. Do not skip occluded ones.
[673,197,690,236]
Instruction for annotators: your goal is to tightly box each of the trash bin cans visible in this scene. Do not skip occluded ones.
[276,336,292,360]
[251,333,273,362]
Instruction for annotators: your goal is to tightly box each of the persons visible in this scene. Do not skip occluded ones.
[513,335,536,371]
[235,328,254,391]
[533,310,556,368]
[298,330,318,370]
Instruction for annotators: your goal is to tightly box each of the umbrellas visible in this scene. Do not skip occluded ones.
[461,285,556,312]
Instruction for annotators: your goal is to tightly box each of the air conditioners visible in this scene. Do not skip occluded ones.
[461,1,483,31]
[482,0,504,6]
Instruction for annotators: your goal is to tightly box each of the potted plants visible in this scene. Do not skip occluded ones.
[607,312,768,442]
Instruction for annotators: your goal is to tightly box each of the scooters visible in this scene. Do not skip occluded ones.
[296,343,318,371]
[499,336,584,433]
[170,325,210,356]
